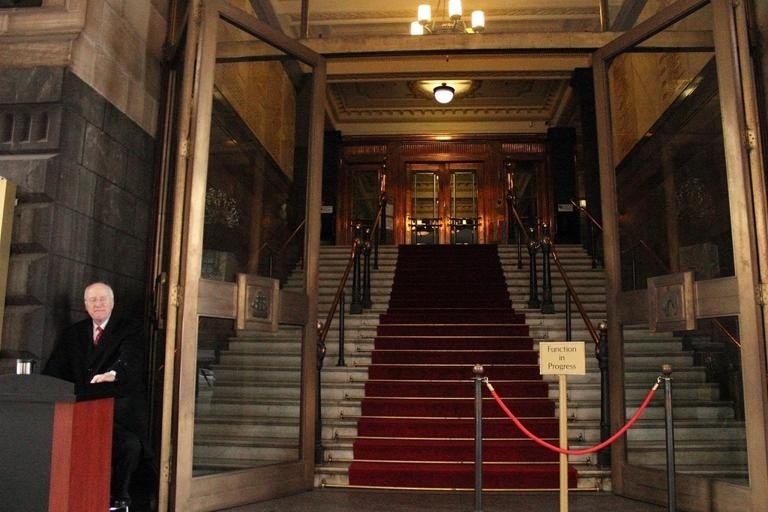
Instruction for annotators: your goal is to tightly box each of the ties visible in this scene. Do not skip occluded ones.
[94,326,104,345]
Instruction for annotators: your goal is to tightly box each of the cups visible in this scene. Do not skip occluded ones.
[15,360,32,376]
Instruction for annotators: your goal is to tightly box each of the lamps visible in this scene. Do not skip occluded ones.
[403,0,486,106]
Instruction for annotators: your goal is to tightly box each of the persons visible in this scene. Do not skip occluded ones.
[42,281,149,512]
